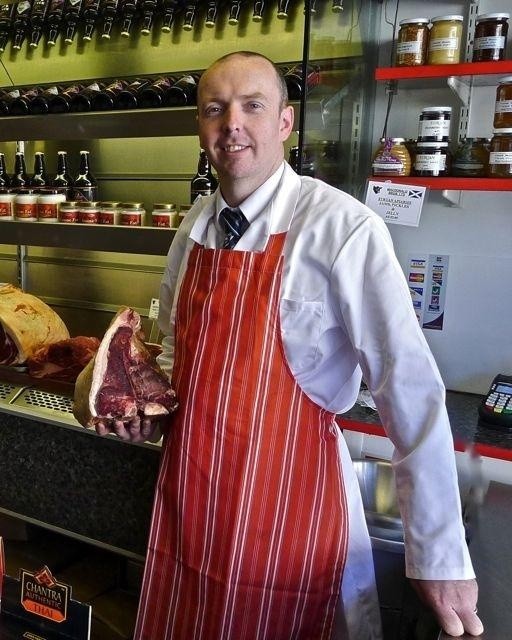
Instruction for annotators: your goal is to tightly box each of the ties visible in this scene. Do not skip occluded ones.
[219,207,247,249]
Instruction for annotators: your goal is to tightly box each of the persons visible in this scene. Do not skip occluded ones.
[96,52,485,640]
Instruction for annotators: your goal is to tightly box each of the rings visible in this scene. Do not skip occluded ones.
[474,608,479,614]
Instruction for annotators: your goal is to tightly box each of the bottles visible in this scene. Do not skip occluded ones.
[0,62,320,117]
[1,139,349,227]
[0,0,344,52]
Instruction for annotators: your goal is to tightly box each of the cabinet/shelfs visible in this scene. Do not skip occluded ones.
[370,60,512,204]
[0,56,362,256]
